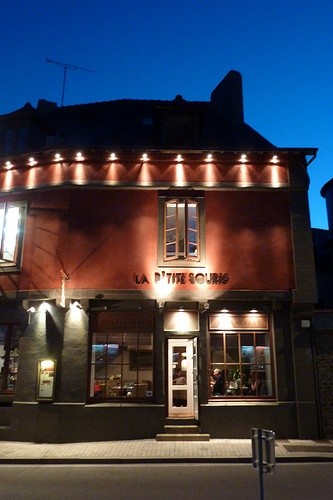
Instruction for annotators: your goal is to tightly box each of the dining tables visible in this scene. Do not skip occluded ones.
[227,385,248,396]
[110,386,134,398]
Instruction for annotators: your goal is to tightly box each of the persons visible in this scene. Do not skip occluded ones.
[172,368,264,396]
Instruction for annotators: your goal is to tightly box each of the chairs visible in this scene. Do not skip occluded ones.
[129,384,148,398]
[99,384,116,397]
